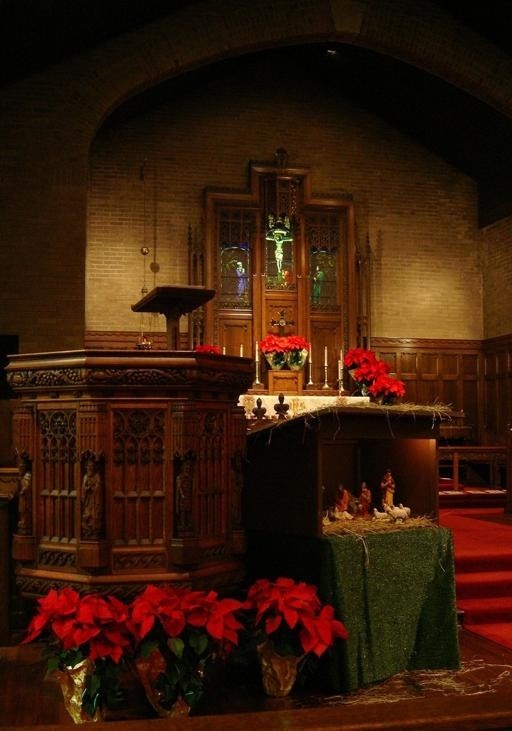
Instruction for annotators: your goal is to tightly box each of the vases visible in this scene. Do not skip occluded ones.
[45,661,102,728]
[139,650,202,716]
[258,638,305,694]
[266,350,307,370]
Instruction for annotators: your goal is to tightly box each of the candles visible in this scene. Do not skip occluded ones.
[223,347,226,355]
[340,350,344,369]
[255,341,259,361]
[324,345,328,365]
[309,343,312,364]
[239,344,244,358]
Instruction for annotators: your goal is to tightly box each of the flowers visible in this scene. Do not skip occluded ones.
[20,587,127,672]
[126,590,243,649]
[343,347,406,404]
[259,334,310,365]
[244,578,349,656]
[195,344,222,356]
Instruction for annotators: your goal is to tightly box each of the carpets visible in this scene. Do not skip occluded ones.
[1,627,511,726]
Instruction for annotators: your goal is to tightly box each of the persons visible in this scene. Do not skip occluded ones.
[334,483,349,512]
[380,470,395,506]
[312,265,325,304]
[235,262,249,297]
[358,481,371,518]
[17,463,32,535]
[265,235,293,273]
[176,462,191,532]
[81,462,102,539]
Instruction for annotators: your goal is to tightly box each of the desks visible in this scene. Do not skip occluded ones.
[438,444,509,491]
